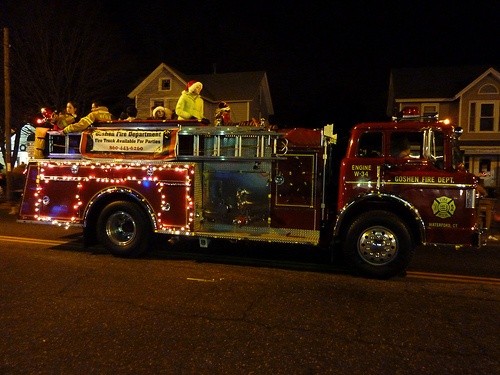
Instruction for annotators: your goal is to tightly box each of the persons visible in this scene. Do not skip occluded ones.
[53,99,78,130]
[118,104,138,120]
[60,101,112,136]
[151,105,165,119]
[175,80,210,125]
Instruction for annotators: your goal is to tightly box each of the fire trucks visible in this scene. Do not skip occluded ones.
[16,106,487,278]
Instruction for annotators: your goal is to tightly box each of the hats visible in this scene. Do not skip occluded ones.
[152,105,166,119]
[181,80,203,95]
